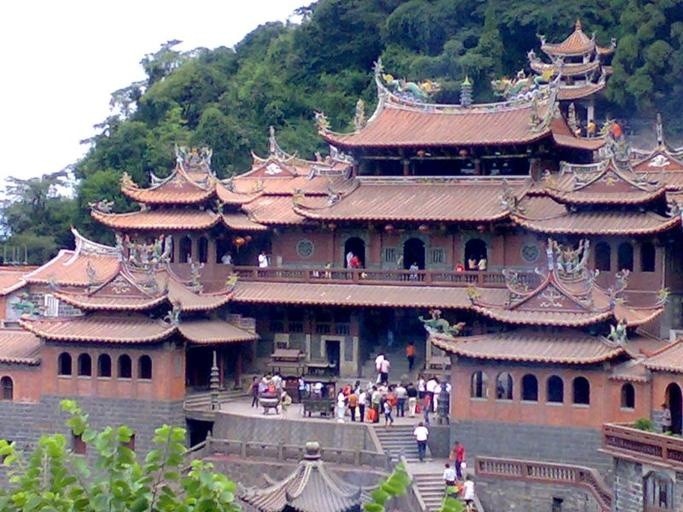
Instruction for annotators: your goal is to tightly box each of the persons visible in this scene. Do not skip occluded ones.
[345,250,353,278]
[405,341,416,372]
[185,251,191,263]
[449,440,465,478]
[414,421,429,461]
[220,252,231,263]
[468,255,476,281]
[461,476,475,512]
[258,251,268,277]
[497,380,504,400]
[245,371,450,430]
[574,125,582,137]
[585,119,596,137]
[613,121,620,142]
[477,255,486,272]
[227,250,235,263]
[443,463,457,497]
[408,261,419,281]
[324,263,331,277]
[380,357,389,386]
[454,260,464,281]
[349,254,362,278]
[374,350,383,384]
[660,403,672,432]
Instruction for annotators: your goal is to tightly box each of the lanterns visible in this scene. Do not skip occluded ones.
[233,236,244,247]
[244,235,251,245]
[459,149,468,160]
[416,150,427,162]
